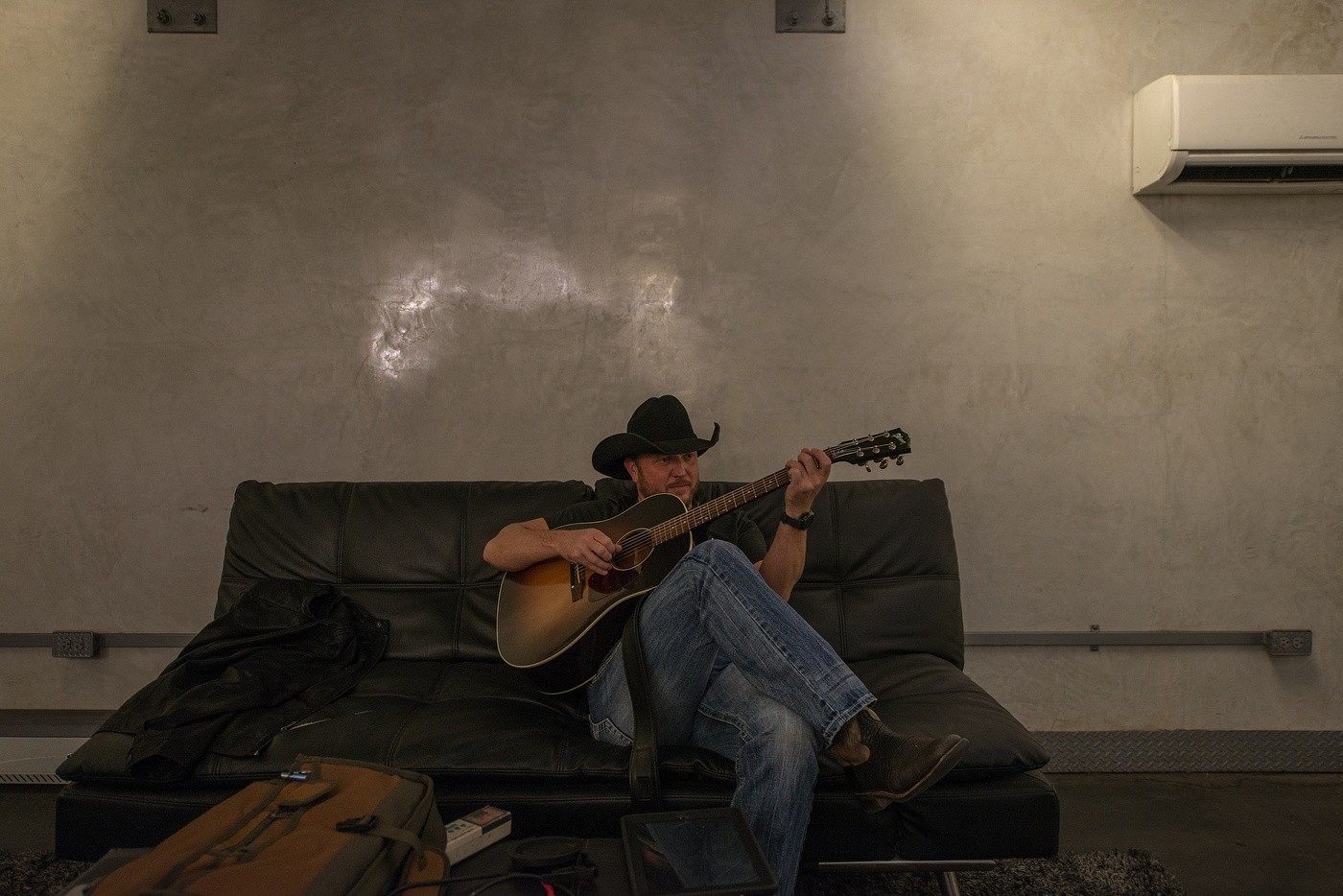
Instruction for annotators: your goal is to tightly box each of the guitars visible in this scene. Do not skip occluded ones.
[493,428,912,696]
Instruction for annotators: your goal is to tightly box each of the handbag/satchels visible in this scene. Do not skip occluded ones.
[80,752,450,895]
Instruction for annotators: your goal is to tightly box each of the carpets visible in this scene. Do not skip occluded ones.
[0,845,1181,895]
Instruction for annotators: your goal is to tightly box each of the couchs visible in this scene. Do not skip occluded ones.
[50,476,1063,896]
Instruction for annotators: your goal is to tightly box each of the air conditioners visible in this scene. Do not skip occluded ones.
[1128,72,1343,194]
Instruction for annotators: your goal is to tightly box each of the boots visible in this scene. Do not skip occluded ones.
[834,708,970,813]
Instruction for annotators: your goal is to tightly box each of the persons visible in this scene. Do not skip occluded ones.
[480,395,969,895]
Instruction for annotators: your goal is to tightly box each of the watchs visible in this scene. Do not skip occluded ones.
[780,505,815,531]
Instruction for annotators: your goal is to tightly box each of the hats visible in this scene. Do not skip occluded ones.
[591,395,721,481]
